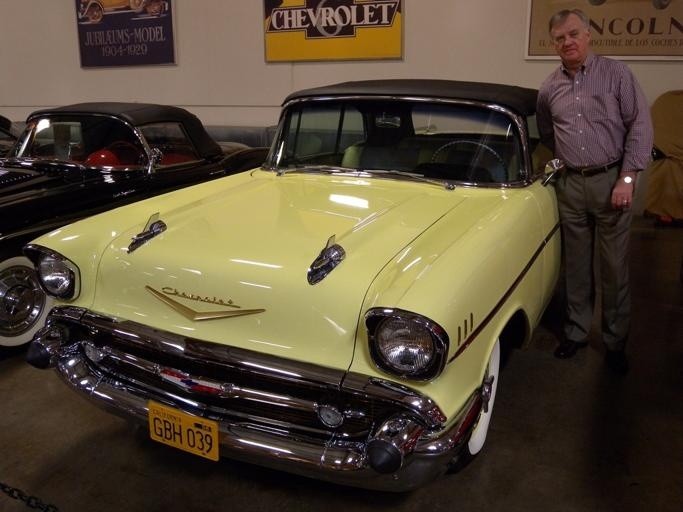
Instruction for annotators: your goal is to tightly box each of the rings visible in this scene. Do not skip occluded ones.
[623,199,627,205]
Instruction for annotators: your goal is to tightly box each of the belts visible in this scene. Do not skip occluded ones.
[581,162,617,177]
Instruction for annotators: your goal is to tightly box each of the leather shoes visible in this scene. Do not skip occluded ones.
[554,339,588,359]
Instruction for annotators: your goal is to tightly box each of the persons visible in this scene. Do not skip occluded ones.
[536,9,655,374]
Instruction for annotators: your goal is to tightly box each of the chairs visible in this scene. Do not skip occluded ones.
[341,146,519,183]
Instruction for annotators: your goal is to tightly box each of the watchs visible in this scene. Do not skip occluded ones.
[619,175,635,184]
